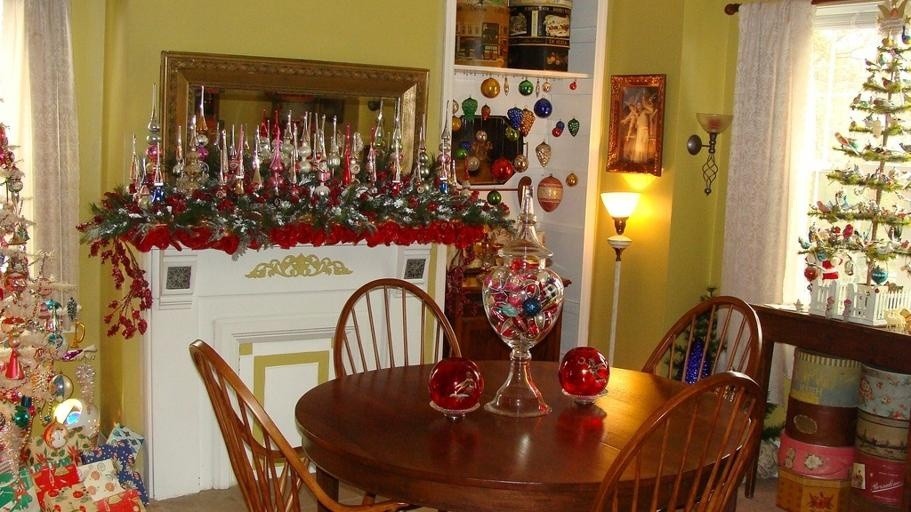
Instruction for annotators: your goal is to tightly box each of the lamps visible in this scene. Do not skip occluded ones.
[687,113,734,196]
[599,193,645,365]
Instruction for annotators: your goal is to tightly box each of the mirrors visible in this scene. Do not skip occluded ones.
[160,51,430,184]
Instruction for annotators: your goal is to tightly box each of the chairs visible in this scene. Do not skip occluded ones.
[590,370,769,511]
[642,294,769,406]
[189,338,410,512]
[332,264,466,380]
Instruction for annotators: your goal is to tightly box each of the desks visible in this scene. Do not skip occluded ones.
[291,357,759,511]
[746,299,911,511]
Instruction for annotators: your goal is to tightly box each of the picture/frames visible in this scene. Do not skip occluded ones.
[609,76,665,176]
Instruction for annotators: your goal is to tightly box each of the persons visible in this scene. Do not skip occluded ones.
[621,94,657,165]
